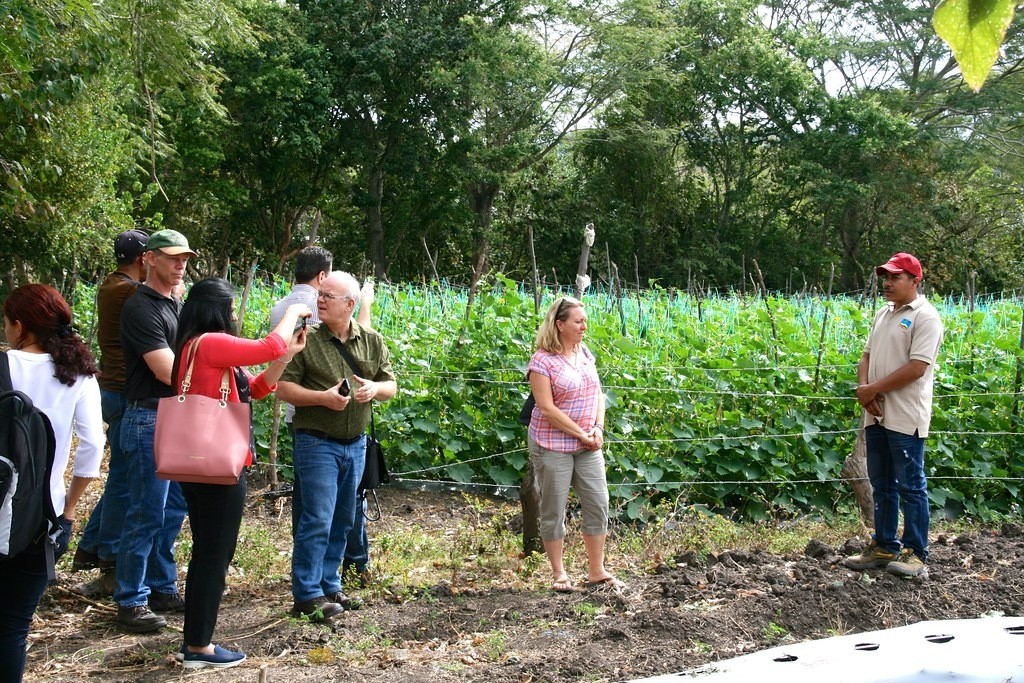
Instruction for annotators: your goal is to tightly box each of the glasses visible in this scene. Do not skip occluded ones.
[555,297,577,320]
[314,291,348,301]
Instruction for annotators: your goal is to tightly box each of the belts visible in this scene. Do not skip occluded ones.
[129,397,158,410]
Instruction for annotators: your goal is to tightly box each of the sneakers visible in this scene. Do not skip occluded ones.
[117,605,167,632]
[331,591,364,608]
[147,591,186,612]
[846,538,896,569]
[888,549,926,576]
[294,596,344,619]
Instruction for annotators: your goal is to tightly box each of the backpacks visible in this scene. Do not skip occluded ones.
[0,351,58,558]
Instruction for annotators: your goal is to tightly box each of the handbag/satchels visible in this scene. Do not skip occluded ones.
[362,436,389,490]
[153,331,249,485]
[520,392,537,425]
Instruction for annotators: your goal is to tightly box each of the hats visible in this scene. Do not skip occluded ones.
[875,253,923,282]
[115,229,150,257]
[147,229,197,256]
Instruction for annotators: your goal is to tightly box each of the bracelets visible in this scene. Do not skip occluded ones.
[594,424,604,432]
[278,358,292,363]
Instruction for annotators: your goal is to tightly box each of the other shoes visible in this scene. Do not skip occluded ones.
[74,546,99,568]
[177,642,247,668]
[99,559,118,571]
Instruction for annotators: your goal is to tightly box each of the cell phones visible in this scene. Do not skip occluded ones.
[338,379,351,396]
[299,317,307,338]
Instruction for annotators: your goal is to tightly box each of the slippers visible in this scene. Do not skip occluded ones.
[584,577,616,587]
[553,580,572,591]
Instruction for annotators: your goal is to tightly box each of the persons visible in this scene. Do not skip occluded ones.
[114,229,199,632]
[271,247,375,581]
[844,252,943,576]
[276,272,397,621]
[0,285,104,683]
[71,228,184,592]
[171,278,312,669]
[526,297,625,591]
